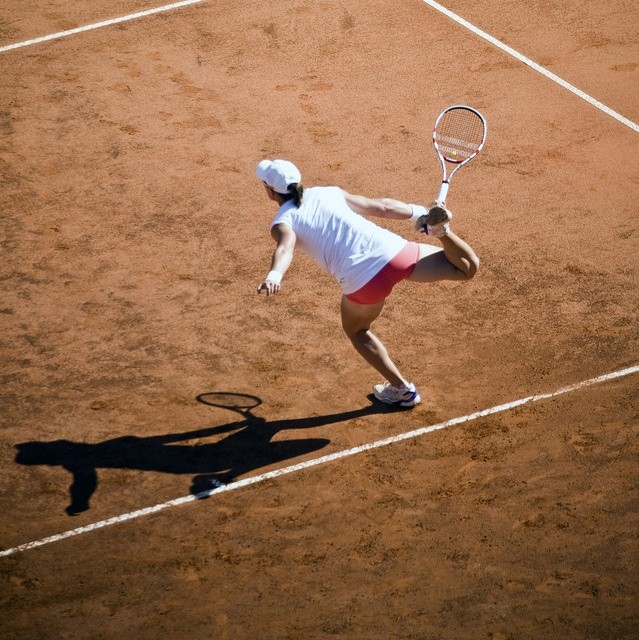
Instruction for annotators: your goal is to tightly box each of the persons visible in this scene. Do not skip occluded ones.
[254,157,481,409]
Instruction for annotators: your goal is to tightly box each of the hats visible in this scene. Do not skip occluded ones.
[255,159,301,194]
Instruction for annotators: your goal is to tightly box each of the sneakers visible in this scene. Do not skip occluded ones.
[373,380,421,406]
[415,206,452,236]
